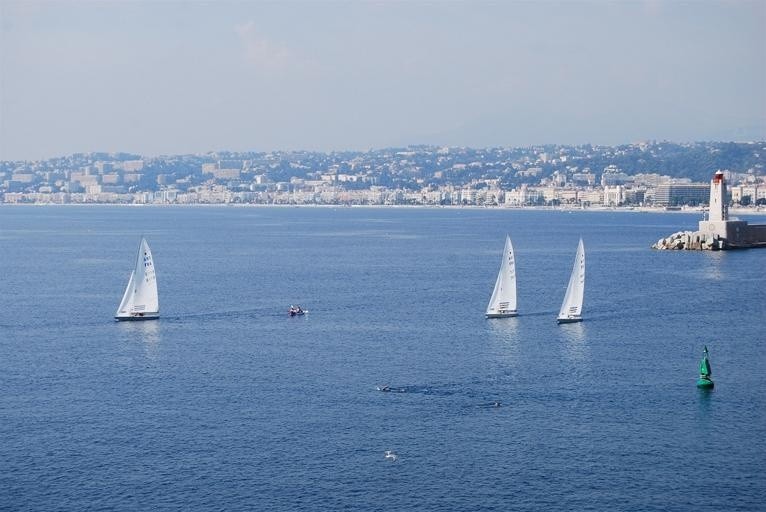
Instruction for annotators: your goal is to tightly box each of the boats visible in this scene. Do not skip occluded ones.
[289,311,303,316]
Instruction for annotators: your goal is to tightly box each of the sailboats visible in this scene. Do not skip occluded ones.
[483,233,519,318]
[556,237,585,324]
[112,234,162,320]
[696,346,714,389]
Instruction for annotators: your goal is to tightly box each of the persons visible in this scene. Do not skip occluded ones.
[288,305,304,317]
